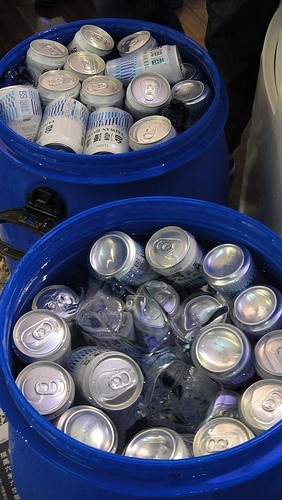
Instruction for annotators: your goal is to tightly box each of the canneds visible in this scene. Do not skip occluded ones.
[0,23,213,155]
[12,226,282,460]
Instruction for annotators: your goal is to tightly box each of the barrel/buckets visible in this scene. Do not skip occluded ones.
[1,19,233,280]
[1,195,281,500]
